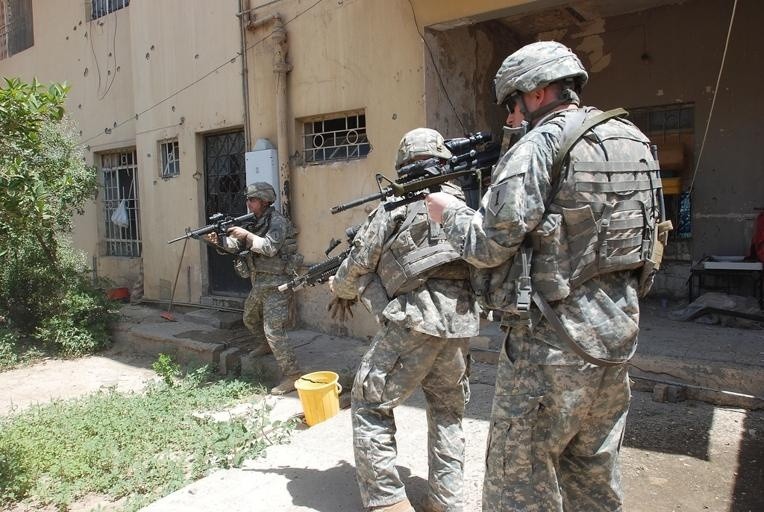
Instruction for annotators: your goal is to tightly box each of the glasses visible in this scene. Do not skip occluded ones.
[505,98,517,114]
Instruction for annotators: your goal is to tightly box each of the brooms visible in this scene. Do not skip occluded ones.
[160,227,190,320]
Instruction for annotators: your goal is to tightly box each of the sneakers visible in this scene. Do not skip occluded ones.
[271,373,302,395]
[250,344,272,358]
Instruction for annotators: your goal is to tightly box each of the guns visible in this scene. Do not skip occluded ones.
[167,212,261,256]
[329,129,501,215]
[278,223,365,293]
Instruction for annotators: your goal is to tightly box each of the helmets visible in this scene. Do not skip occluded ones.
[247,182,276,204]
[395,128,454,168]
[494,41,589,105]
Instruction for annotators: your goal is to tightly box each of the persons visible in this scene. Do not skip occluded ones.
[423,36,673,512]
[207,183,302,394]
[325,127,481,512]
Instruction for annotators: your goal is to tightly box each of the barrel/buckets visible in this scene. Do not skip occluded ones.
[294,370,343,427]
